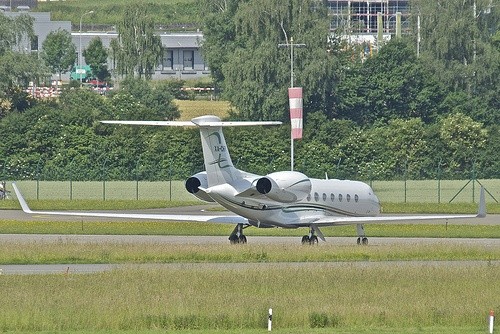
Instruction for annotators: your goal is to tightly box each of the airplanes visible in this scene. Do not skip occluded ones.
[10,114,486,246]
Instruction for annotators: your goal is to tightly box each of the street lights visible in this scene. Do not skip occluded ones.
[79,11,95,85]
